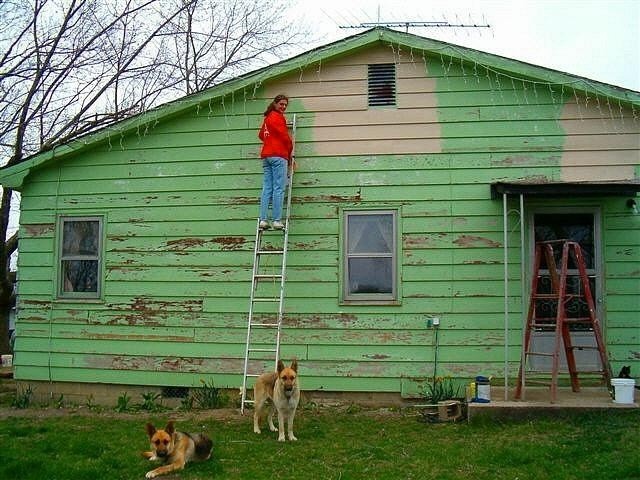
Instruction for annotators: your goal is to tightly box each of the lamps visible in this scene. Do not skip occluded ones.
[627,199,638,215]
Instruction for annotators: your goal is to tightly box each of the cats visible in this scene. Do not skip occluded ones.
[618,366,640,390]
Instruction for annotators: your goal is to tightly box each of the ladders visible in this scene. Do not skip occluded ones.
[241,115,296,416]
[515,239,616,403]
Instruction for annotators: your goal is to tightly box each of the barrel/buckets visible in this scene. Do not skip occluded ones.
[1,355,13,368]
[610,378,635,404]
[475,376,490,403]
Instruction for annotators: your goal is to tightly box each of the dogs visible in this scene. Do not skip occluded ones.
[141,418,214,479]
[253,358,300,442]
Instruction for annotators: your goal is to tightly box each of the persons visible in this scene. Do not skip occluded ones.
[257,94,294,232]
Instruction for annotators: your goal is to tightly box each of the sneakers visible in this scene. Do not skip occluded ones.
[273,221,284,229]
[259,220,271,228]
